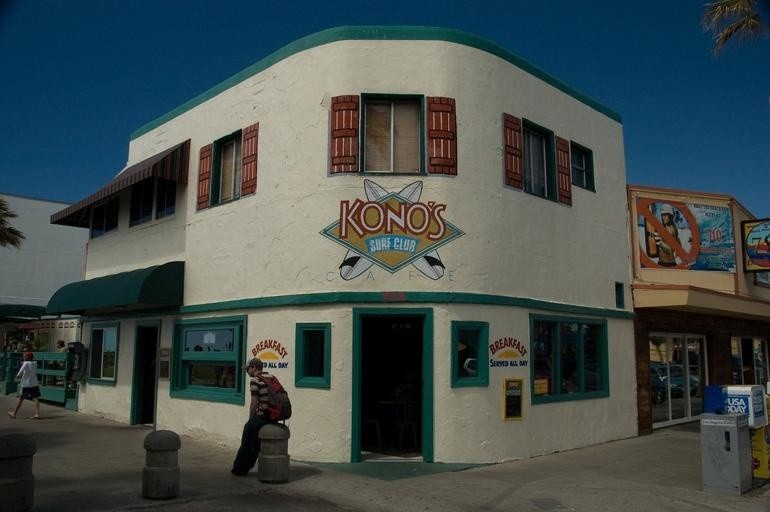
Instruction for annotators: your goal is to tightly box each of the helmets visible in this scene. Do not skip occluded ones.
[660,203,674,217]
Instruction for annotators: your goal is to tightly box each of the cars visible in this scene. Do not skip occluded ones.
[650,360,700,402]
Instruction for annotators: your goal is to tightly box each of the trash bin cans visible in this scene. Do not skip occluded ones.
[700,413,753,496]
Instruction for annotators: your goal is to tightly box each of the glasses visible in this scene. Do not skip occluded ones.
[247,368,249,372]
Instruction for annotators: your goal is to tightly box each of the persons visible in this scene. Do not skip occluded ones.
[5,350,42,421]
[0,330,40,352]
[54,339,70,369]
[653,204,682,265]
[231,357,279,476]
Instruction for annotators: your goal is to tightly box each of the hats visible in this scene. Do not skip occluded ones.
[56,340,64,346]
[248,358,263,369]
[24,352,33,360]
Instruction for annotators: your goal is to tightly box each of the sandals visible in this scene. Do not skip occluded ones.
[8,412,16,418]
[30,416,40,420]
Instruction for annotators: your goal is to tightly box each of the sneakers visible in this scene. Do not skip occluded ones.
[231,468,248,476]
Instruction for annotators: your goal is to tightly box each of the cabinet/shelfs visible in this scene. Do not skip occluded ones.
[17,352,70,403]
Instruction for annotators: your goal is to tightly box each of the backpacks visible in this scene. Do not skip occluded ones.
[254,374,292,421]
